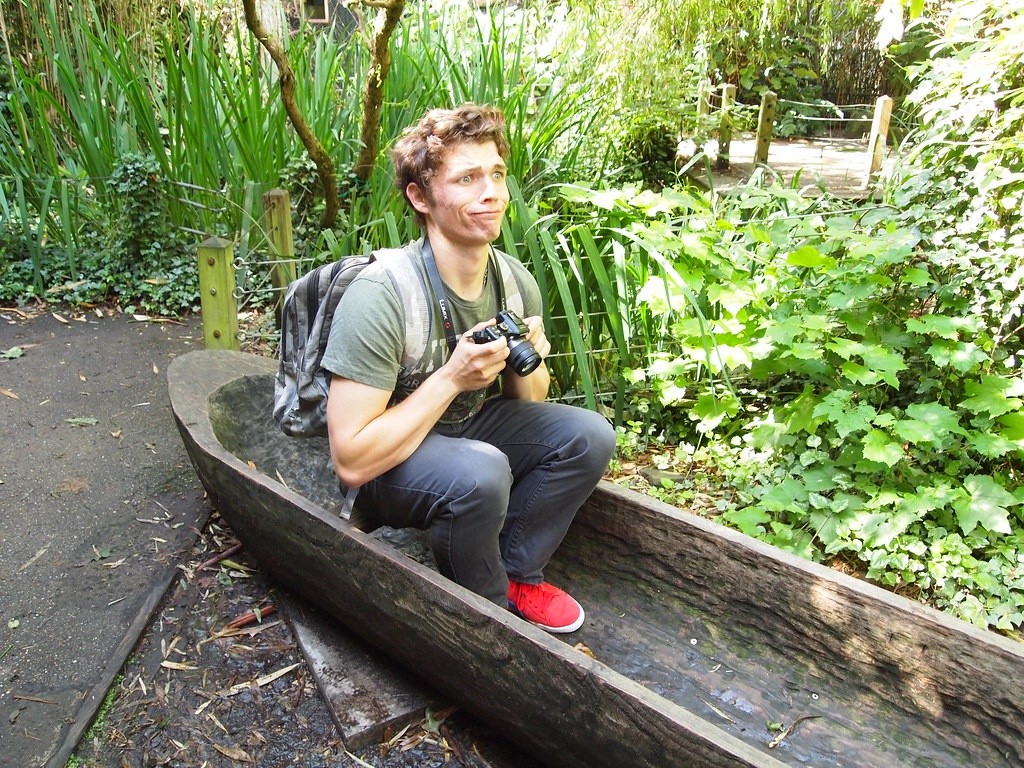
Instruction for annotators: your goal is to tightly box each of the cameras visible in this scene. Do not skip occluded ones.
[474,310,542,378]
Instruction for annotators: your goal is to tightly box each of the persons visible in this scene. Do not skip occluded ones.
[323,102,616,636]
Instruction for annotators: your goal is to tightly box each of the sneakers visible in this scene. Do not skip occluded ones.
[505,577,585,633]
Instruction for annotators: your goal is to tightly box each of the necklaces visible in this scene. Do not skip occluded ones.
[483,264,488,290]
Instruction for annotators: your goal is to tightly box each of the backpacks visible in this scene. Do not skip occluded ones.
[272,247,525,439]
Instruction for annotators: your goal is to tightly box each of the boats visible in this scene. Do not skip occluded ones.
[165,346,1023,767]
